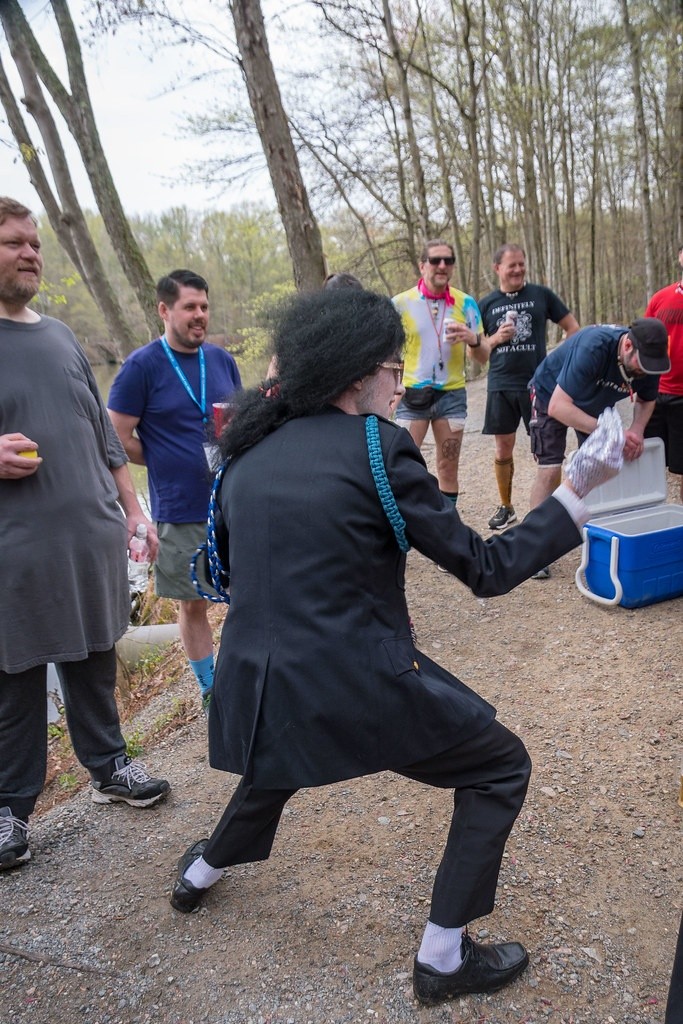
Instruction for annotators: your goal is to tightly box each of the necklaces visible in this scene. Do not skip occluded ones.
[618,336,634,403]
[432,299,438,320]
[425,301,445,370]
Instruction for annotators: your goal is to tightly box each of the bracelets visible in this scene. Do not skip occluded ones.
[469,334,481,348]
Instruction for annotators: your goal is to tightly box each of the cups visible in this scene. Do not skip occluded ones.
[443,318,456,343]
[506,311,518,334]
[213,403,239,438]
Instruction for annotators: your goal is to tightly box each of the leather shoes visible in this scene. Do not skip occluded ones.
[410,931,529,1006]
[170,837,212,913]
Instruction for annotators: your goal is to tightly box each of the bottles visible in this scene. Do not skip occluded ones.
[127,525,151,593]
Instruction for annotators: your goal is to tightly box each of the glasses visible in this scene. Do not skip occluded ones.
[377,360,405,383]
[422,256,455,265]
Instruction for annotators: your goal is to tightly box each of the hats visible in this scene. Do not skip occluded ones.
[628,317,672,373]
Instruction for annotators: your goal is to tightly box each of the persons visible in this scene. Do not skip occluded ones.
[107,269,242,719]
[269,274,361,379]
[173,288,624,1004]
[0,196,173,871]
[528,317,670,578]
[391,239,488,573]
[476,243,581,531]
[644,246,683,488]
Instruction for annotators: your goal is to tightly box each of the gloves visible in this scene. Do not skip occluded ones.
[566,408,626,499]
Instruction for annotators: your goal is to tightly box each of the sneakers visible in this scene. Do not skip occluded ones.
[0,806,33,868]
[91,755,172,808]
[489,505,517,530]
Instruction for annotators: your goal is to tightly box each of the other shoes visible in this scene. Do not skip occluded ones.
[531,566,549,578]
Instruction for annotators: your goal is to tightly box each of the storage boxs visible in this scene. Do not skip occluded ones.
[569,437,683,609]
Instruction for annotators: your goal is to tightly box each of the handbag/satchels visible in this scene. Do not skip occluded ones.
[403,387,447,410]
